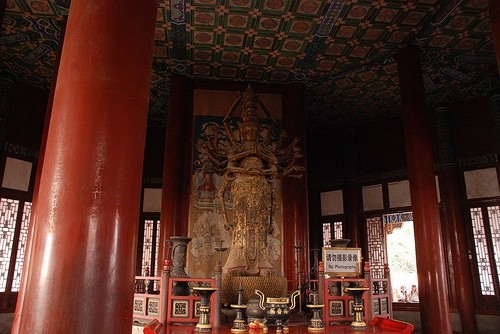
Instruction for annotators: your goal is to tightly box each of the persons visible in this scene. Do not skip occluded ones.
[195,103,306,275]
[397,285,418,302]
